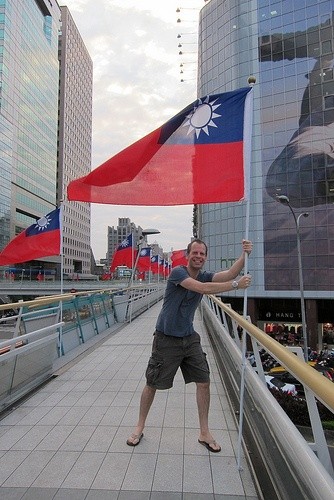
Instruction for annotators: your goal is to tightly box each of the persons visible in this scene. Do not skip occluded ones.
[127,239,253,454]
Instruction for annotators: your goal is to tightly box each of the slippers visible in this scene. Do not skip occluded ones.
[198,438,221,453]
[126,432,144,446]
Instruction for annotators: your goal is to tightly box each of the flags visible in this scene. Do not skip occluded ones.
[110,234,189,277]
[0,208,60,265]
[67,87,252,206]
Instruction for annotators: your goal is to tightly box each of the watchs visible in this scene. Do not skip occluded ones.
[232,280,238,290]
